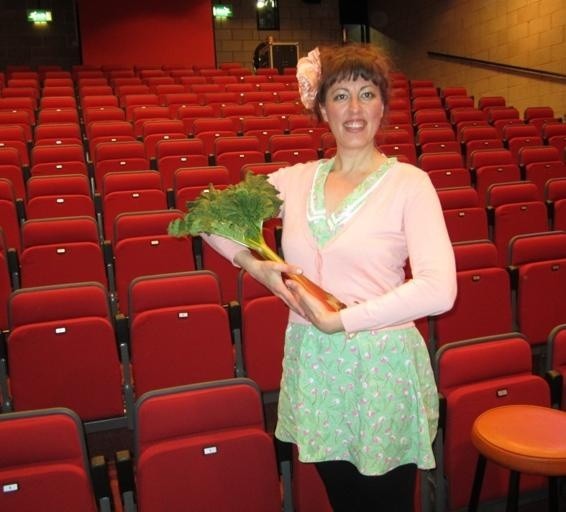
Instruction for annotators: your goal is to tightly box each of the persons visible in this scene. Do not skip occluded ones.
[194,45,457,512]
